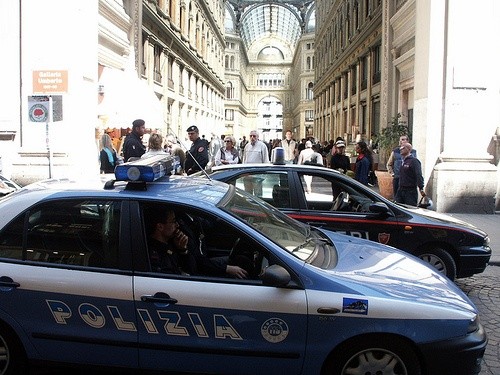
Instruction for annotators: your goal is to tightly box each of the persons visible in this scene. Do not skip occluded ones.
[99,120,428,211]
[146,201,255,279]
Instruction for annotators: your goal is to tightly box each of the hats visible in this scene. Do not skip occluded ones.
[132,119,145,126]
[306,141,312,147]
[336,140,345,148]
[186,126,197,132]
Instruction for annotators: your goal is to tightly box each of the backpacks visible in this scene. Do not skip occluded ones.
[368,169,377,186]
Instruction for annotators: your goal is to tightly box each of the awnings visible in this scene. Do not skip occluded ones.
[98,67,166,129]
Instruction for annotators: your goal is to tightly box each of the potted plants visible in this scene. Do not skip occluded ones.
[372,113,411,199]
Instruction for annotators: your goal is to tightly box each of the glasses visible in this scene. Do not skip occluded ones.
[250,135,256,137]
[400,146,405,149]
[166,218,179,223]
[224,140,232,143]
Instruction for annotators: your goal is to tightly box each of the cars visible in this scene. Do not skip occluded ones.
[179,161,492,284]
[0,146,488,375]
